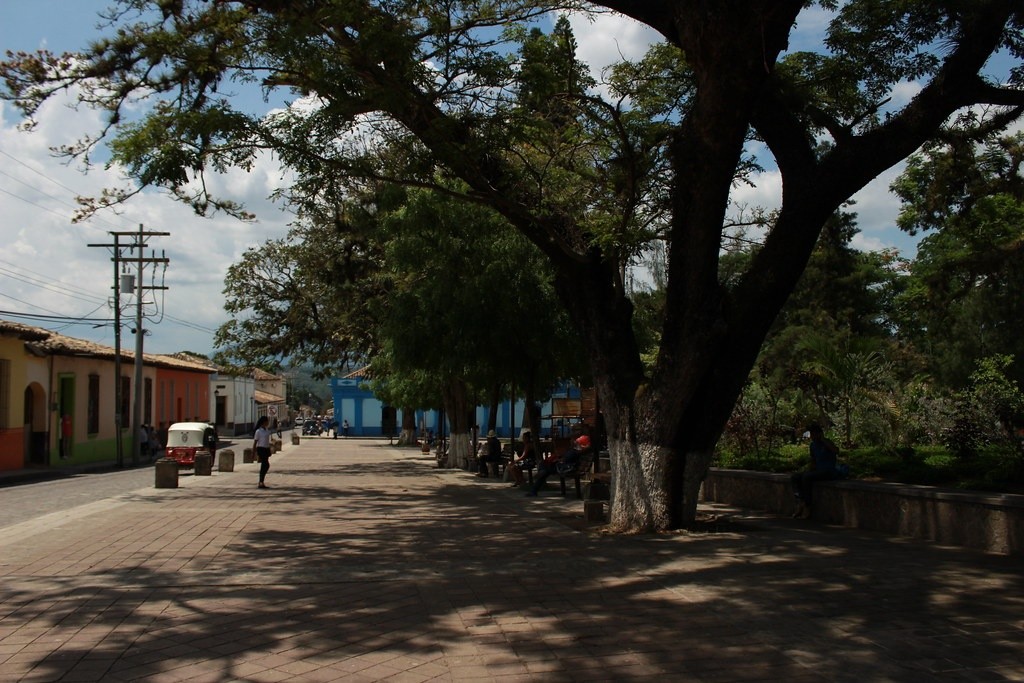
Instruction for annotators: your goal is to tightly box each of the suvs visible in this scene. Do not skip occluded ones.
[302,419,318,435]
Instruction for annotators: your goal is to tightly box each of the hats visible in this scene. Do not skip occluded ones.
[486,430,496,438]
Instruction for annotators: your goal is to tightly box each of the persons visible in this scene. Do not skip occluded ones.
[790,423,839,519]
[428,430,434,444]
[251,416,271,488]
[508,420,600,497]
[273,417,283,438]
[140,424,158,458]
[318,418,349,439]
[57,413,73,459]
[476,430,501,478]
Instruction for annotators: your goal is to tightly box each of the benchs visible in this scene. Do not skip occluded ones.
[496,444,517,474]
[559,453,594,498]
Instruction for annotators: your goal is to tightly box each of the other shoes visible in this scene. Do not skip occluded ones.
[526,492,536,496]
[793,507,803,518]
[477,473,488,477]
[258,485,269,488]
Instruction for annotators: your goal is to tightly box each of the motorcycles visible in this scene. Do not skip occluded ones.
[165,422,217,468]
